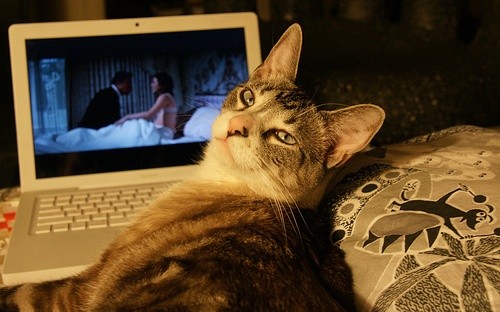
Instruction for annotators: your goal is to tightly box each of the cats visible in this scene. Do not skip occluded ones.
[0,21,388,312]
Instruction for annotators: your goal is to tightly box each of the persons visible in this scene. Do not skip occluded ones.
[114,72,178,138]
[78,69,132,130]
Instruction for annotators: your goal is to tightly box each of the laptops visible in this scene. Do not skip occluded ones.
[1,10,263,287]
